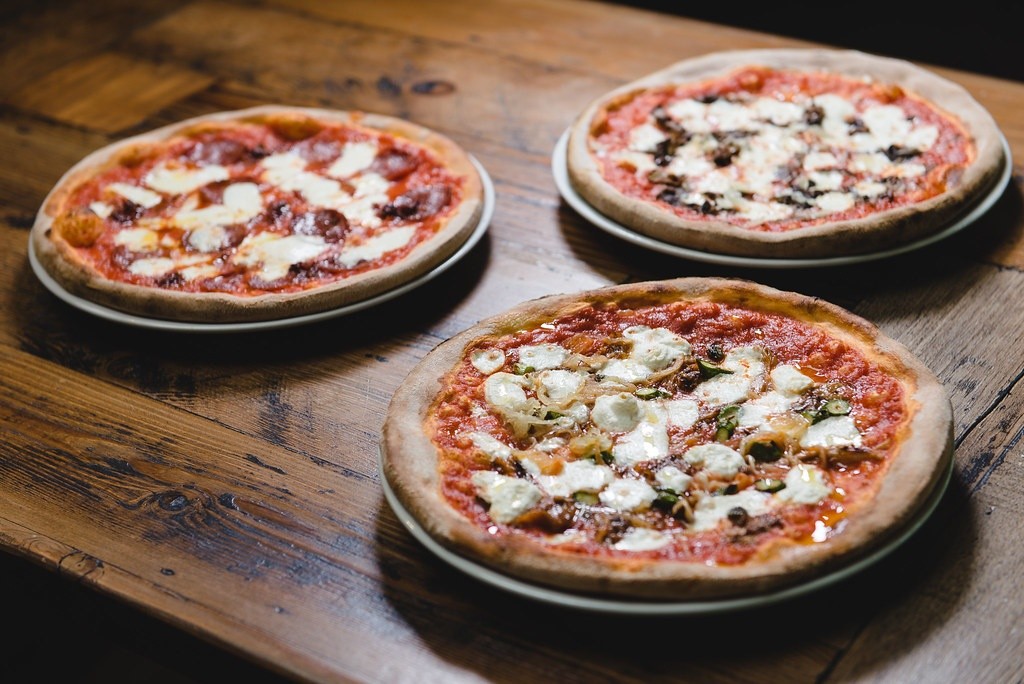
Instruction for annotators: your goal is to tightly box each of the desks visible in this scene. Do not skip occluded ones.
[0,0,1024,684]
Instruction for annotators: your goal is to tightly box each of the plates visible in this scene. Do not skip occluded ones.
[551,129,1011,267]
[376,448,960,616]
[26,152,497,332]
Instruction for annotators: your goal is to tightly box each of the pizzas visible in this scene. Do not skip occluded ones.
[565,46,1006,257]
[31,103,486,323]
[376,274,954,601]
